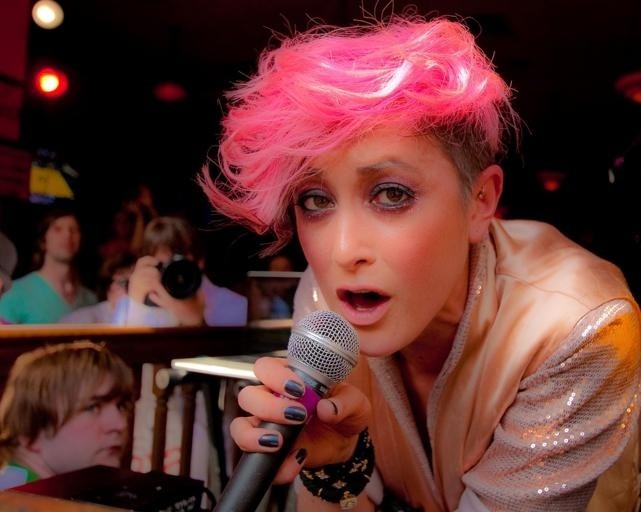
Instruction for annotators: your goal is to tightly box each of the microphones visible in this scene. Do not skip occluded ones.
[211,309,361,512]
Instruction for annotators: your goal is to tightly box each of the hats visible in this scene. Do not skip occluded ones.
[39,209,79,240]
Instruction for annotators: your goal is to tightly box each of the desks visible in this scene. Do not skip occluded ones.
[171,346,293,488]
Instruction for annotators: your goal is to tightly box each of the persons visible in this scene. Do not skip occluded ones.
[251,252,291,319]
[105,217,248,327]
[196,0,640,511]
[0,342,134,493]
[0,211,97,325]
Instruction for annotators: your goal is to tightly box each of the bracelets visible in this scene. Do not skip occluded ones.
[298,427,377,509]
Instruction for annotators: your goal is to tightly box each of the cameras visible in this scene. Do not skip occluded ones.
[154,257,204,298]
